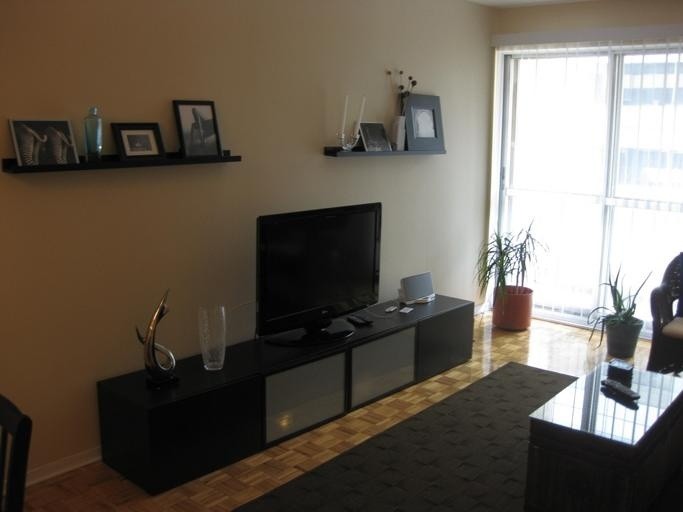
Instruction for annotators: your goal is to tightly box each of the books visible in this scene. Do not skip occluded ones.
[396,271,436,306]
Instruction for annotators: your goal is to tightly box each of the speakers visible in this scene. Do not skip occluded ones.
[398,272,435,305]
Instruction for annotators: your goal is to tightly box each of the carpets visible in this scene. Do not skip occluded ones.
[233,361,577,512]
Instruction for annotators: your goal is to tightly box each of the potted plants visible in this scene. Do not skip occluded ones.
[587,264,653,358]
[474,217,545,330]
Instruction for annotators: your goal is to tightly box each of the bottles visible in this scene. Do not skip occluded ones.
[83,105,102,163]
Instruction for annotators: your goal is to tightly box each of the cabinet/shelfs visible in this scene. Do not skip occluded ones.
[96,293,474,495]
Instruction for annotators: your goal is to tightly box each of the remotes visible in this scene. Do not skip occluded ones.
[350,313,374,323]
[347,316,365,325]
[601,379,640,400]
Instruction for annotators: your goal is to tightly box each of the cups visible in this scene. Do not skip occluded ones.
[197,304,225,372]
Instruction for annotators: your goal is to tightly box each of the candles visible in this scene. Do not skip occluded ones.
[341,95,349,132]
[354,97,367,136]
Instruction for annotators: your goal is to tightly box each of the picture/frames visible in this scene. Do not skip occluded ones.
[359,122,392,152]
[9,119,80,166]
[173,100,223,160]
[403,93,444,150]
[110,122,168,162]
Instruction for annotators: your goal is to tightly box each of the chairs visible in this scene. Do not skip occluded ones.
[0,394,32,512]
[646,252,683,378]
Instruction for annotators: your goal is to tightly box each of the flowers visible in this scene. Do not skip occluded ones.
[387,70,417,151]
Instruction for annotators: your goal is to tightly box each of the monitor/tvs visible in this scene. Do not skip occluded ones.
[255,202,382,350]
